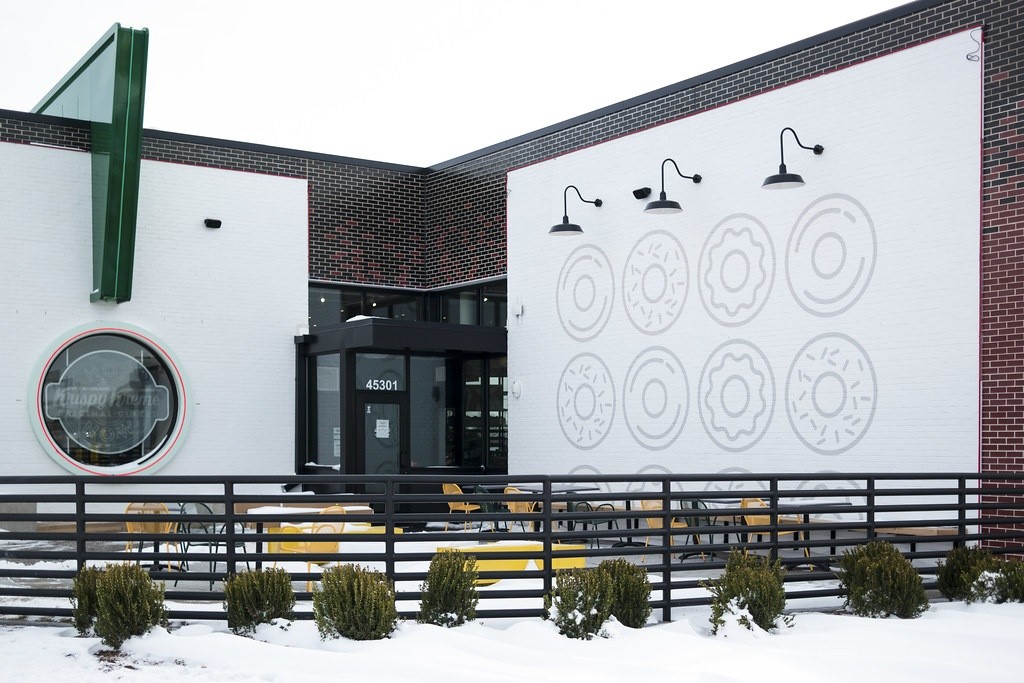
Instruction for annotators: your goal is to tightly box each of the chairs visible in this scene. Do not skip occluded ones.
[272,506,346,592]
[124,502,189,570]
[442,483,494,532]
[641,500,705,562]
[680,498,740,563]
[740,498,813,571]
[566,492,624,549]
[174,503,250,591]
[504,487,560,544]
[474,485,526,532]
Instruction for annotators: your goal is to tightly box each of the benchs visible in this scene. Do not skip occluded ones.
[437,543,585,584]
[501,501,967,555]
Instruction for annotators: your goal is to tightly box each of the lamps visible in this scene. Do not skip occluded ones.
[761,127,824,190]
[643,158,703,214]
[547,185,603,236]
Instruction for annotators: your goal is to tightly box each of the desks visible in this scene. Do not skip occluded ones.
[516,485,600,532]
[462,485,516,532]
[777,503,852,571]
[128,507,186,572]
